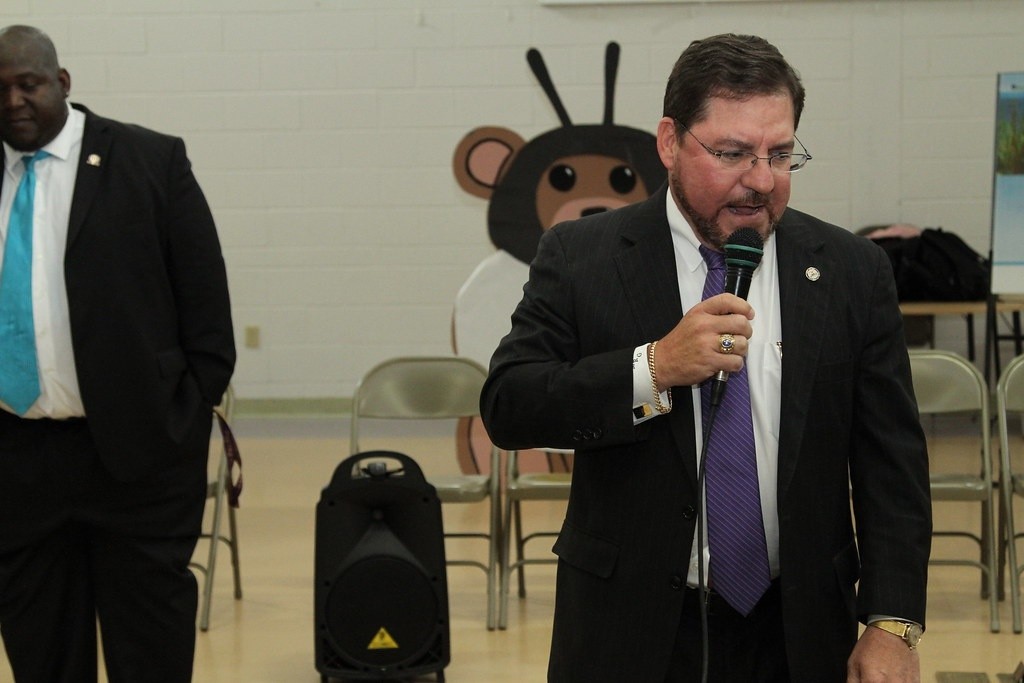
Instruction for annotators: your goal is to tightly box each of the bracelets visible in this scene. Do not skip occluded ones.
[650,341,673,413]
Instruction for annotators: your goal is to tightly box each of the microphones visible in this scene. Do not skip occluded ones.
[710,226,764,405]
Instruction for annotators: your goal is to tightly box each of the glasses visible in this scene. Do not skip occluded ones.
[673,118,812,174]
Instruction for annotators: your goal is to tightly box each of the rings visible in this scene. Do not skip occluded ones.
[719,334,735,353]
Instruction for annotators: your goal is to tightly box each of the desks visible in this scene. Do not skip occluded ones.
[897,294,1024,387]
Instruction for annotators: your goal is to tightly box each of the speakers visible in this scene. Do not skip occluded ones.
[313,450,448,683]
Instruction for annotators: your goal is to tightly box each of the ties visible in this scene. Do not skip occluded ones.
[698,243,773,619]
[0,148,53,418]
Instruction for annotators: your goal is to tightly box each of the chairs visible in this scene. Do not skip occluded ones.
[901,348,1000,634]
[183,384,242,630]
[496,443,575,631]
[349,352,504,631]
[993,353,1024,634]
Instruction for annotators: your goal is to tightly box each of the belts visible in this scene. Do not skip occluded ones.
[679,589,784,616]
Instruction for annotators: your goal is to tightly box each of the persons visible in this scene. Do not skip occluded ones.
[0,25,236,683]
[479,34,932,683]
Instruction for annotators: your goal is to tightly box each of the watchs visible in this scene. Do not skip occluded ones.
[871,620,922,650]
